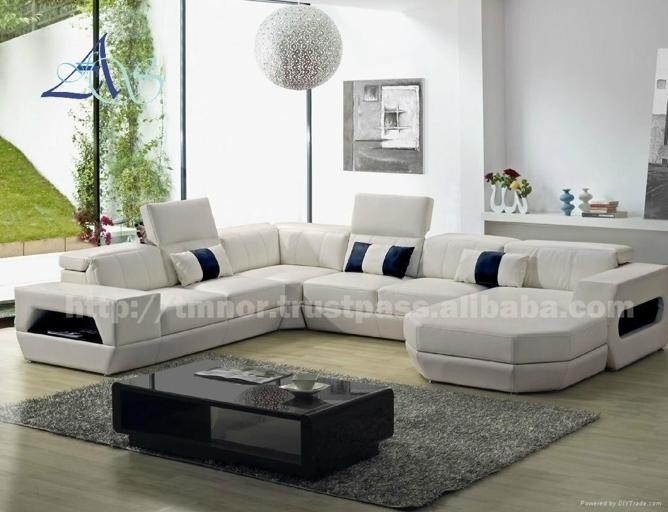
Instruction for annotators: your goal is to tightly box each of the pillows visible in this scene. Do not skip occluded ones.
[454,248,529,287]
[169,244,233,287]
[343,242,415,280]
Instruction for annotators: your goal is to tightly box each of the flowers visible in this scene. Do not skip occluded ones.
[509,179,532,198]
[484,168,520,199]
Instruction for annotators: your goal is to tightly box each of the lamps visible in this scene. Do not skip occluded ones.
[253,2,344,90]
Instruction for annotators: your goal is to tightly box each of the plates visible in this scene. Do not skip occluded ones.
[279,382,331,398]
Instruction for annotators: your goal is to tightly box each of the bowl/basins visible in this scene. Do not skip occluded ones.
[292,373,319,390]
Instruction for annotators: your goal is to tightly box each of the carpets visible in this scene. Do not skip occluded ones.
[0,351,600,508]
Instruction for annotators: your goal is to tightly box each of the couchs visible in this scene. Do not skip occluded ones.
[14,194,667,392]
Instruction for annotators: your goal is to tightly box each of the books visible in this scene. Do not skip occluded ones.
[582,200,629,218]
[195,366,292,384]
[47,329,84,340]
